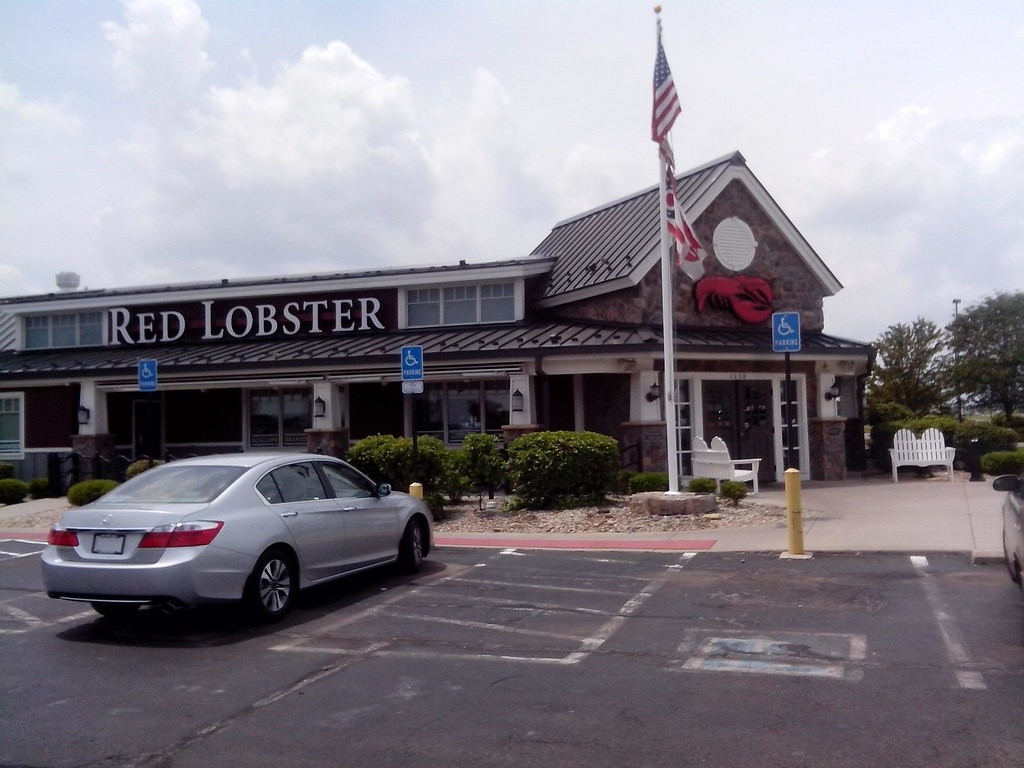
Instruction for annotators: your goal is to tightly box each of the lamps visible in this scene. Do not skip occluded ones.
[77,405,89,424]
[314,396,325,416]
[646,382,660,402]
[511,388,523,412]
[824,380,841,401]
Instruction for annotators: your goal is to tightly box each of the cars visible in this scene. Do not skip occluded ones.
[39,450,436,626]
[993,473,1024,593]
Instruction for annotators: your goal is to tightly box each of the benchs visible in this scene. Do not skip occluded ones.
[888,427,956,482]
[691,435,762,496]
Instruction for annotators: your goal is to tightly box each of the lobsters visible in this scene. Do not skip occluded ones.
[691,273,775,325]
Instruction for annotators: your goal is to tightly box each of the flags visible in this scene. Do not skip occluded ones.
[664,162,707,281]
[650,34,681,170]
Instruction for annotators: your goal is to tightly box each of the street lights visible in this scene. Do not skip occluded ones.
[952,299,962,421]
